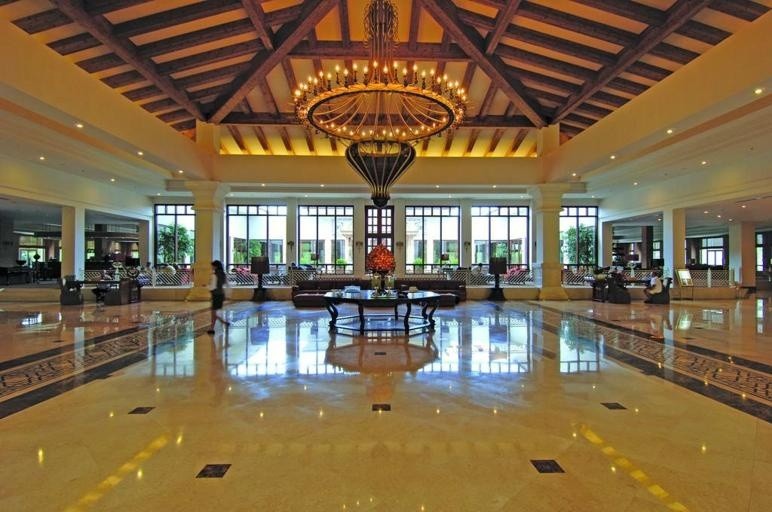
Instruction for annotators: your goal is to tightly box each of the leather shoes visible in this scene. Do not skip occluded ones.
[206,329,215,335]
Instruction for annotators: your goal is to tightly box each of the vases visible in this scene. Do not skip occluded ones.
[378,272,387,293]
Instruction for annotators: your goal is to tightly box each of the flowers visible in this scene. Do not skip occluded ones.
[366,243,397,272]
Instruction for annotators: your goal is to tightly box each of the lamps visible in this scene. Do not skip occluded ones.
[490,301,507,343]
[293,0,470,150]
[250,302,269,344]
[251,256,272,301]
[488,256,507,300]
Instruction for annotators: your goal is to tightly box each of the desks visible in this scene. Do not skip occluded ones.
[323,329,439,374]
[69,280,121,293]
[323,290,441,336]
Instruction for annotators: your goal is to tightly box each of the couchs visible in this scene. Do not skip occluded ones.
[291,279,370,307]
[394,279,466,302]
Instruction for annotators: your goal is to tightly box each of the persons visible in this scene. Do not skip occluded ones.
[643,271,662,304]
[202,260,231,334]
[470,263,482,285]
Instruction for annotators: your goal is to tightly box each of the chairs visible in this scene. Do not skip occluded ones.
[104,277,131,305]
[59,278,83,305]
[565,270,584,285]
[653,278,672,304]
[505,268,525,285]
[607,276,630,304]
[173,268,191,285]
[232,268,254,286]
[283,266,305,286]
